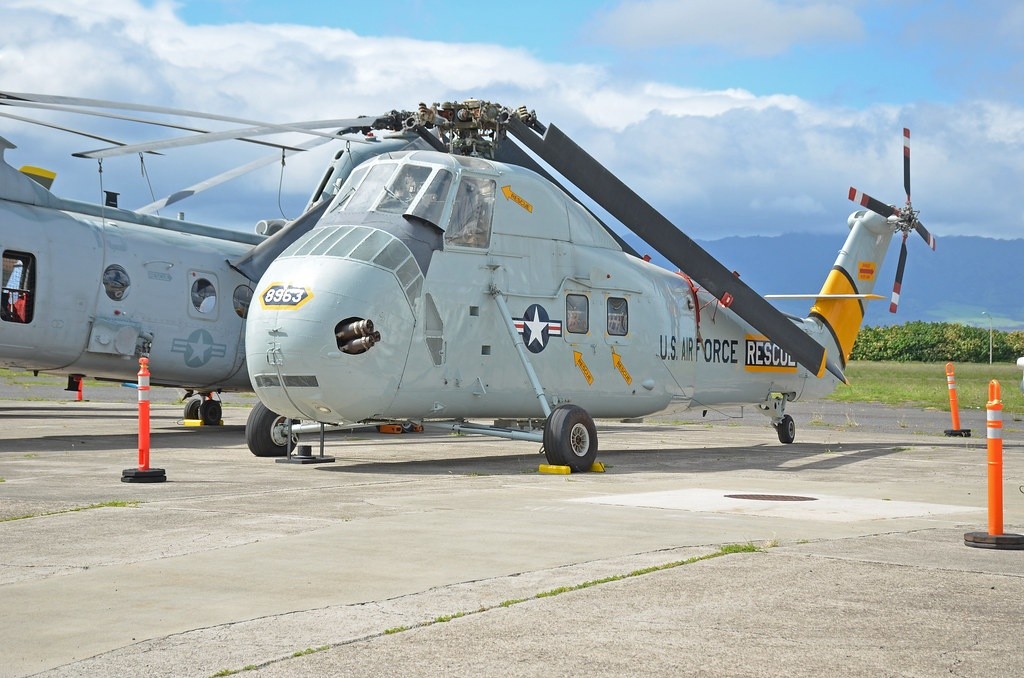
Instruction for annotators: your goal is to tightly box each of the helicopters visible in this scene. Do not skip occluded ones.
[0,91,938,476]
[0,137,296,425]
